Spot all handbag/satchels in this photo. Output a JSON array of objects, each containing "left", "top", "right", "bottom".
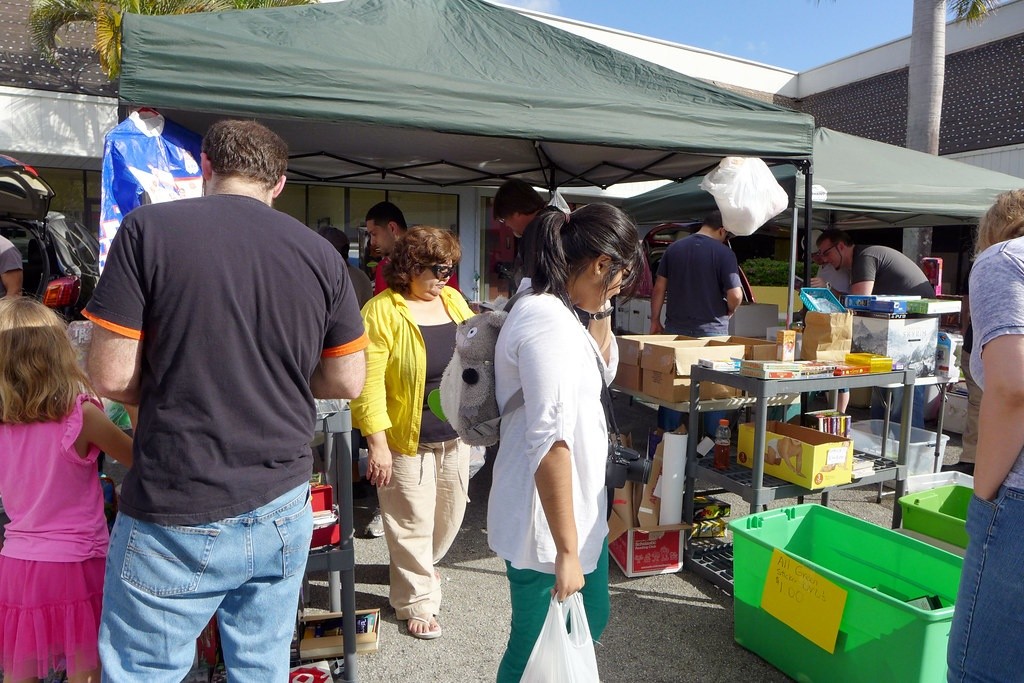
[{"left": 518, "top": 590, "right": 604, "bottom": 683}]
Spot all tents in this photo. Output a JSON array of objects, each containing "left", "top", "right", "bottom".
[
  {"left": 119, "top": 2, "right": 814, "bottom": 522},
  {"left": 618, "top": 124, "right": 1024, "bottom": 507}
]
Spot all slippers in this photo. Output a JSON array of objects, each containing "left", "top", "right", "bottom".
[{"left": 407, "top": 612, "right": 442, "bottom": 639}]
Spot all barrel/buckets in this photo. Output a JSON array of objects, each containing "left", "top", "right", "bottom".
[{"left": 924, "top": 383, "right": 943, "bottom": 421}]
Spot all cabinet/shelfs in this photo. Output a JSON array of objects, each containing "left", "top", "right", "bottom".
[
  {"left": 104, "top": 403, "right": 361, "bottom": 683},
  {"left": 684, "top": 364, "right": 916, "bottom": 597}
]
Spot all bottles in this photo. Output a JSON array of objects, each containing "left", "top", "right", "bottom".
[{"left": 713, "top": 419, "right": 731, "bottom": 469}]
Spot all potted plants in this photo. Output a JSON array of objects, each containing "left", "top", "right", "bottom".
[{"left": 741, "top": 259, "right": 819, "bottom": 314}]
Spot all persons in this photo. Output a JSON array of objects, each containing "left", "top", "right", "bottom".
[
  {"left": 946, "top": 189, "right": 1024, "bottom": 683},
  {"left": 809, "top": 228, "right": 941, "bottom": 430},
  {"left": 0, "top": 117, "right": 642, "bottom": 683},
  {"left": 650, "top": 214, "right": 745, "bottom": 443},
  {"left": 801, "top": 229, "right": 851, "bottom": 301}
]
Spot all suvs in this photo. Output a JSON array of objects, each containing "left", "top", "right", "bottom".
[{"left": 0, "top": 153, "right": 98, "bottom": 322}]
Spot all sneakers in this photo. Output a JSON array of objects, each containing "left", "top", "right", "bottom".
[{"left": 364, "top": 511, "right": 385, "bottom": 537}]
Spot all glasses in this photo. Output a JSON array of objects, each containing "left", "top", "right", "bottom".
[
  {"left": 819, "top": 241, "right": 838, "bottom": 257},
  {"left": 618, "top": 261, "right": 636, "bottom": 290},
  {"left": 498, "top": 214, "right": 509, "bottom": 223}
]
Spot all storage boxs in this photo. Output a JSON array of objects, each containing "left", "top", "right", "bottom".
[
  {"left": 310, "top": 485, "right": 341, "bottom": 548},
  {"left": 614, "top": 258, "right": 975, "bottom": 683}
]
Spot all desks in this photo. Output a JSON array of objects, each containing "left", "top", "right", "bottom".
[
  {"left": 877, "top": 375, "right": 965, "bottom": 504},
  {"left": 609, "top": 383, "right": 802, "bottom": 426}
]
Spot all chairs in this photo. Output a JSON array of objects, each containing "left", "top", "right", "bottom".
[
  {"left": 138, "top": 107, "right": 159, "bottom": 115},
  {"left": 28, "top": 239, "right": 43, "bottom": 286}
]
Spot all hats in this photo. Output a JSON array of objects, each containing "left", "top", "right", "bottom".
[{"left": 419, "top": 264, "right": 455, "bottom": 279}]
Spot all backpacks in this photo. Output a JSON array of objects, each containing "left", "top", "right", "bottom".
[{"left": 438, "top": 287, "right": 563, "bottom": 446}]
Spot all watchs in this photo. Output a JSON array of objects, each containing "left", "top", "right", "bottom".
[{"left": 588, "top": 306, "right": 613, "bottom": 320}]
